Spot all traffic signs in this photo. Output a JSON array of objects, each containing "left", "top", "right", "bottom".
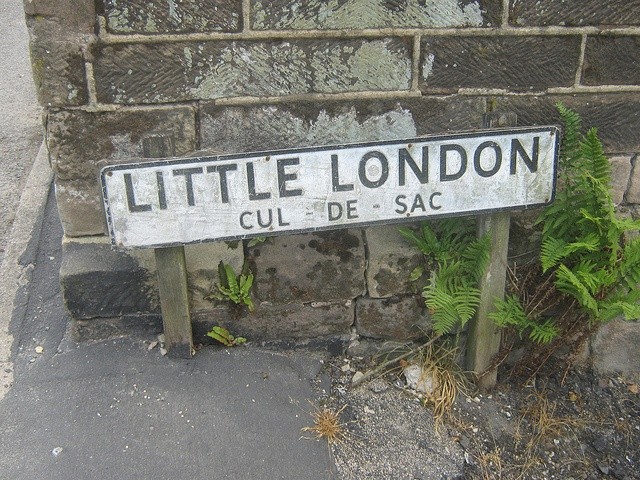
[{"left": 99, "top": 125, "right": 561, "bottom": 252}]
